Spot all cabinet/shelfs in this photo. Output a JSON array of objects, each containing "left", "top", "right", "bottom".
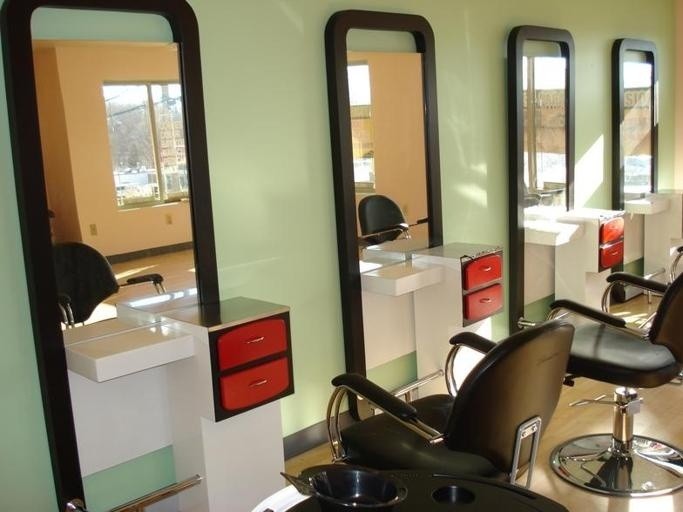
[
  {"left": 167, "top": 300, "right": 299, "bottom": 424},
  {"left": 564, "top": 210, "right": 627, "bottom": 276},
  {"left": 414, "top": 242, "right": 507, "bottom": 329}
]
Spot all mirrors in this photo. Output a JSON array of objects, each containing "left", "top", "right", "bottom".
[
  {"left": 507, "top": 22, "right": 577, "bottom": 229},
  {"left": 0, "top": 0, "right": 222, "bottom": 349},
  {"left": 610, "top": 37, "right": 661, "bottom": 209},
  {"left": 325, "top": 7, "right": 445, "bottom": 273}
]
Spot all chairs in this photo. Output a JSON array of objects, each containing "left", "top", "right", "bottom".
[
  {"left": 324, "top": 317, "right": 579, "bottom": 490},
  {"left": 520, "top": 268, "right": 683, "bottom": 499},
  {"left": 49, "top": 242, "right": 167, "bottom": 331},
  {"left": 358, "top": 195, "right": 430, "bottom": 247}
]
